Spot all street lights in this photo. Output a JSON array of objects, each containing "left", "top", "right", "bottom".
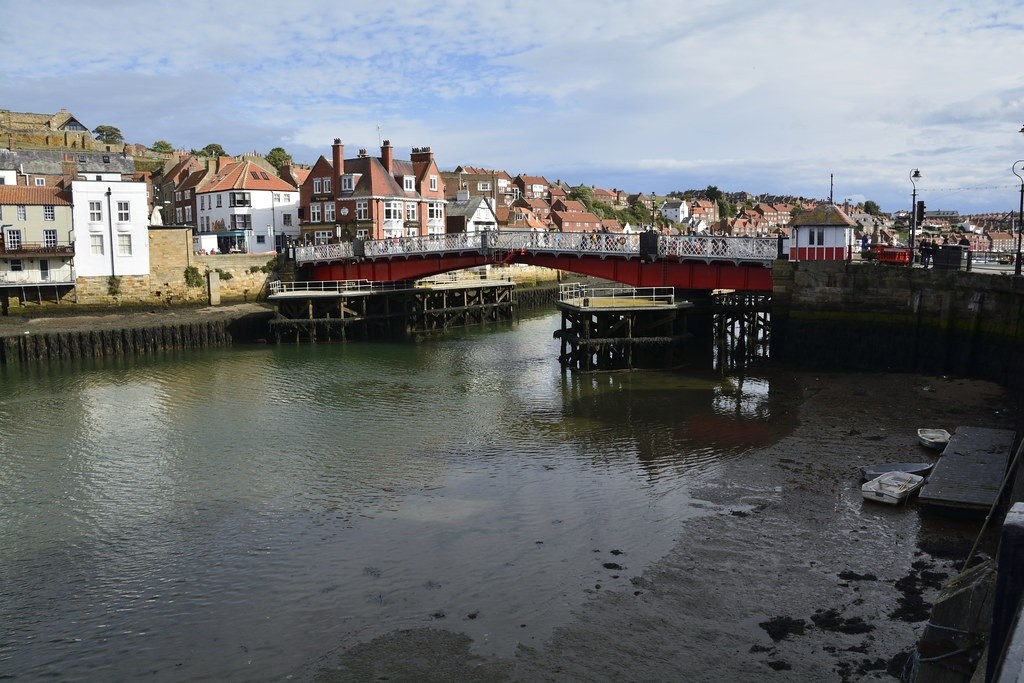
[
  {"left": 651, "top": 192, "right": 656, "bottom": 230},
  {"left": 910, "top": 168, "right": 922, "bottom": 262},
  {"left": 1012, "top": 160, "right": 1024, "bottom": 275}
]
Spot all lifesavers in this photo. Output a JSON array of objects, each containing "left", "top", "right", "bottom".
[
  {"left": 490, "top": 234, "right": 500, "bottom": 247},
  {"left": 618, "top": 237, "right": 626, "bottom": 246}
]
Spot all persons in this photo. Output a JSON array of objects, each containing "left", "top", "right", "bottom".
[
  {"left": 862, "top": 232, "right": 868, "bottom": 251},
  {"left": 530, "top": 227, "right": 621, "bottom": 251},
  {"left": 918, "top": 235, "right": 970, "bottom": 268},
  {"left": 300, "top": 234, "right": 398, "bottom": 259}
]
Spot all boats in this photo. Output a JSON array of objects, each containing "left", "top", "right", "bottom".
[
  {"left": 862, "top": 471, "right": 924, "bottom": 505},
  {"left": 918, "top": 428, "right": 951, "bottom": 448},
  {"left": 859, "top": 463, "right": 934, "bottom": 481}
]
